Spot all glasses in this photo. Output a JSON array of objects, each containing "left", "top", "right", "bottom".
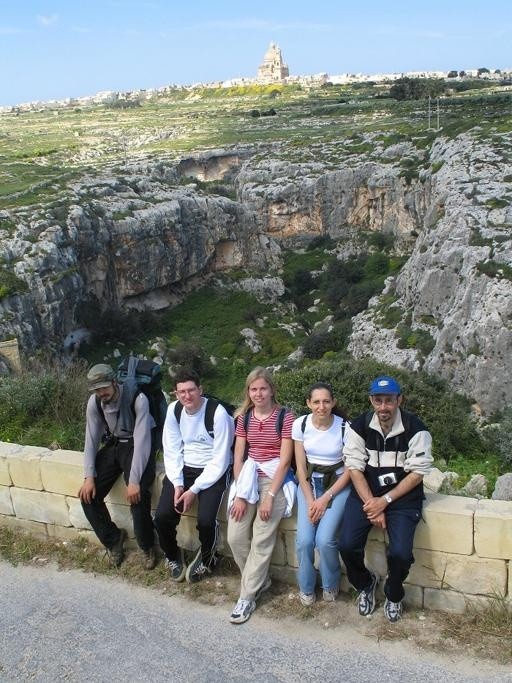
[{"left": 373, "top": 398, "right": 398, "bottom": 405}]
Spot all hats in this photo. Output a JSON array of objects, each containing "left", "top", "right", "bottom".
[
  {"left": 368, "top": 377, "right": 401, "bottom": 396},
  {"left": 87, "top": 363, "right": 117, "bottom": 392}
]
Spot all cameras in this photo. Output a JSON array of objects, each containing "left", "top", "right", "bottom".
[{"left": 378, "top": 472, "right": 397, "bottom": 488}]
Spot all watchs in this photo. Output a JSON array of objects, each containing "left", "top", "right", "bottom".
[
  {"left": 268, "top": 490, "right": 276, "bottom": 498},
  {"left": 384, "top": 494, "right": 393, "bottom": 503},
  {"left": 328, "top": 491, "right": 334, "bottom": 498}
]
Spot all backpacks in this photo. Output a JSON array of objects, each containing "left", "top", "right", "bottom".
[
  {"left": 174, "top": 394, "right": 237, "bottom": 438},
  {"left": 95, "top": 356, "right": 167, "bottom": 450}
]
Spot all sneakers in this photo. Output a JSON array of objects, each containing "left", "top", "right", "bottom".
[
  {"left": 229, "top": 599, "right": 257, "bottom": 624},
  {"left": 322, "top": 584, "right": 341, "bottom": 601},
  {"left": 108, "top": 527, "right": 128, "bottom": 567},
  {"left": 299, "top": 589, "right": 317, "bottom": 607},
  {"left": 185, "top": 546, "right": 218, "bottom": 584},
  {"left": 141, "top": 542, "right": 156, "bottom": 570},
  {"left": 382, "top": 574, "right": 403, "bottom": 623},
  {"left": 166, "top": 545, "right": 187, "bottom": 582},
  {"left": 356, "top": 570, "right": 381, "bottom": 616},
  {"left": 254, "top": 575, "right": 273, "bottom": 602}
]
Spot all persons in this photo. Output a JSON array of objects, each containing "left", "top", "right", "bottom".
[
  {"left": 155, "top": 370, "right": 235, "bottom": 584},
  {"left": 291, "top": 384, "right": 353, "bottom": 606},
  {"left": 342, "top": 377, "right": 434, "bottom": 622},
  {"left": 78, "top": 363, "right": 157, "bottom": 570},
  {"left": 226, "top": 366, "right": 296, "bottom": 624}
]
